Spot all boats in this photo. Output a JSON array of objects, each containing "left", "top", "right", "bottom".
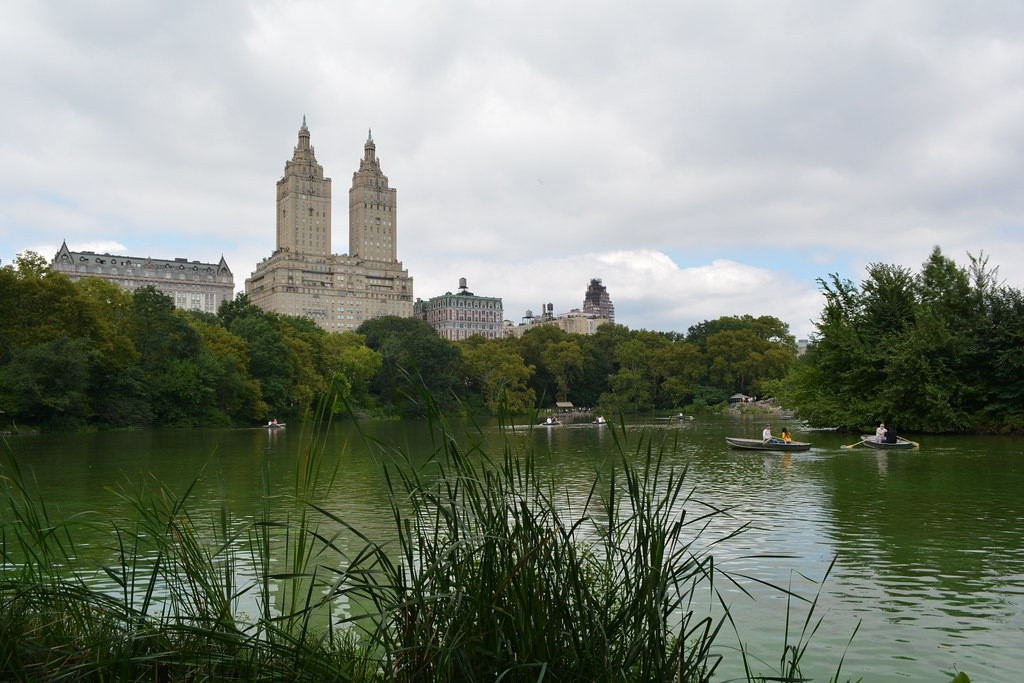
[
  {"left": 860, "top": 433, "right": 916, "bottom": 450},
  {"left": 543, "top": 420, "right": 563, "bottom": 426},
  {"left": 592, "top": 418, "right": 612, "bottom": 424},
  {"left": 262, "top": 423, "right": 288, "bottom": 427},
  {"left": 724, "top": 436, "right": 811, "bottom": 450}
]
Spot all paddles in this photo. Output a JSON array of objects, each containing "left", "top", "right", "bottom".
[
  {"left": 897, "top": 436, "right": 920, "bottom": 447},
  {"left": 761, "top": 437, "right": 772, "bottom": 446},
  {"left": 846, "top": 438, "right": 874, "bottom": 449}
]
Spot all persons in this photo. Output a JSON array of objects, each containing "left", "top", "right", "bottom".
[
  {"left": 781, "top": 428, "right": 791, "bottom": 441},
  {"left": 881, "top": 424, "right": 896, "bottom": 443},
  {"left": 547, "top": 416, "right": 557, "bottom": 424},
  {"left": 875, "top": 424, "right": 887, "bottom": 442},
  {"left": 763, "top": 424, "right": 777, "bottom": 443},
  {"left": 269, "top": 419, "right": 277, "bottom": 425}
]
